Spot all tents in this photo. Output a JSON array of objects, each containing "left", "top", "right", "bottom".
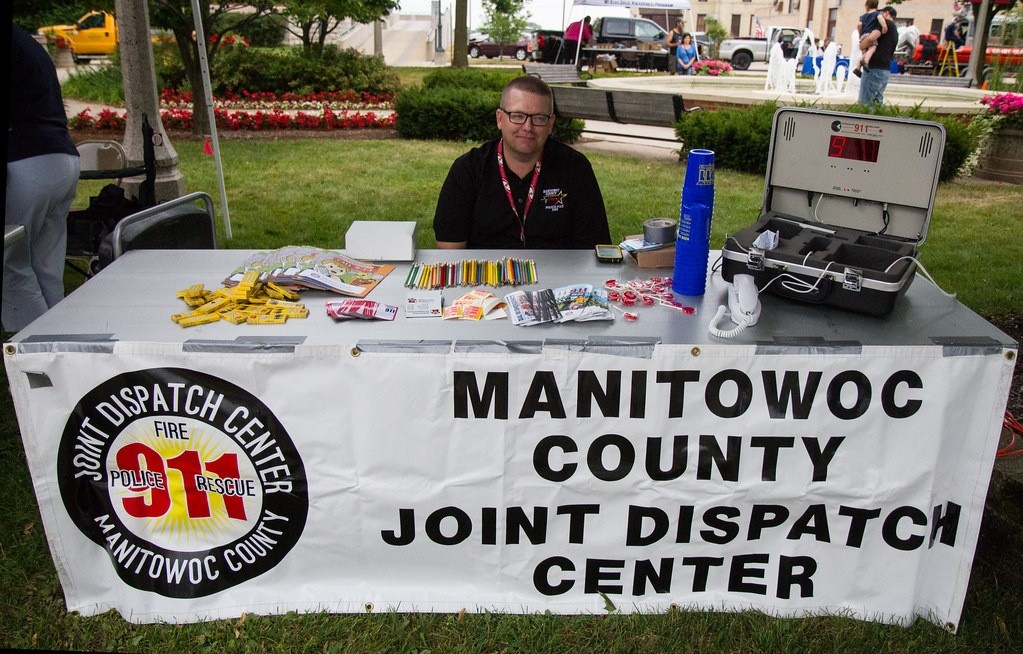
[{"left": 555, "top": 0, "right": 700, "bottom": 66}]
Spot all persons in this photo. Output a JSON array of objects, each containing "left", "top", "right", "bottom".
[
  {"left": 853, "top": 0, "right": 888, "bottom": 77},
  {"left": 561, "top": 295, "right": 586, "bottom": 310},
  {"left": 565, "top": 16, "right": 593, "bottom": 65},
  {"left": 432, "top": 77, "right": 611, "bottom": 250},
  {"left": 1, "top": 23, "right": 81, "bottom": 333},
  {"left": 807, "top": 38, "right": 830, "bottom": 55},
  {"left": 666, "top": 17, "right": 687, "bottom": 75},
  {"left": 944, "top": 22, "right": 963, "bottom": 55},
  {"left": 920, "top": 35, "right": 938, "bottom": 66},
  {"left": 676, "top": 32, "right": 697, "bottom": 76},
  {"left": 957, "top": 27, "right": 965, "bottom": 46},
  {"left": 897, "top": 59, "right": 905, "bottom": 74},
  {"left": 859, "top": 6, "right": 899, "bottom": 105},
  {"left": 790, "top": 34, "right": 801, "bottom": 58}
]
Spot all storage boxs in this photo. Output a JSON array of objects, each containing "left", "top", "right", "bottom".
[
  {"left": 627, "top": 235, "right": 679, "bottom": 269},
  {"left": 638, "top": 40, "right": 662, "bottom": 50},
  {"left": 594, "top": 59, "right": 618, "bottom": 74},
  {"left": 344, "top": 218, "right": 418, "bottom": 265}
]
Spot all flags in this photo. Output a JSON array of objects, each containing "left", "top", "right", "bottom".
[{"left": 755, "top": 18, "right": 762, "bottom": 37}]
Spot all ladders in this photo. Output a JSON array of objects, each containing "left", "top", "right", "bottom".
[{"left": 932, "top": 40, "right": 960, "bottom": 77}]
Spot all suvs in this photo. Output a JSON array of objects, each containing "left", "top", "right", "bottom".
[{"left": 912, "top": 12, "right": 1023, "bottom": 67}]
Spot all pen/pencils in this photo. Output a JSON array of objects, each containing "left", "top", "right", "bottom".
[{"left": 404, "top": 256, "right": 538, "bottom": 291}]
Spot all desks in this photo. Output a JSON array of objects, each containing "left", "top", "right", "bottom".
[
  {"left": 11, "top": 247, "right": 1018, "bottom": 636},
  {"left": 578, "top": 47, "right": 669, "bottom": 74}
]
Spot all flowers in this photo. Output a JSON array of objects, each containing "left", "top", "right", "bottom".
[{"left": 980, "top": 92, "right": 1023, "bottom": 128}]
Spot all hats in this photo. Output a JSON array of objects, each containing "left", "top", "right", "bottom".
[
  {"left": 676, "top": 17, "right": 687, "bottom": 24},
  {"left": 878, "top": 6, "right": 897, "bottom": 18}
]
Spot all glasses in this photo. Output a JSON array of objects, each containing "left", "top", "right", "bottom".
[{"left": 497, "top": 106, "right": 553, "bottom": 126}]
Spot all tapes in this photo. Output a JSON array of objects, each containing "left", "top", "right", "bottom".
[{"left": 643, "top": 218, "right": 677, "bottom": 243}]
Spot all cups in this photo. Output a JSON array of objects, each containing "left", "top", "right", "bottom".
[{"left": 672, "top": 149, "right": 713, "bottom": 296}]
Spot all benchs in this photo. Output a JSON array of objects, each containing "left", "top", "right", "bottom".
[{"left": 521, "top": 63, "right": 591, "bottom": 88}]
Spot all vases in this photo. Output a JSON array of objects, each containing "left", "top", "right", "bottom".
[{"left": 970, "top": 130, "right": 1023, "bottom": 183}]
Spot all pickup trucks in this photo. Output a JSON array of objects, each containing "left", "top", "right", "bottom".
[{"left": 718, "top": 26, "right": 821, "bottom": 71}]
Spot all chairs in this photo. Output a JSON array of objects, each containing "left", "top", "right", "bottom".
[
  {"left": 65, "top": 138, "right": 126, "bottom": 281},
  {"left": 65, "top": 111, "right": 157, "bottom": 279}
]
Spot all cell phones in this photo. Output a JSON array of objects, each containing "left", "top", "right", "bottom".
[{"left": 596, "top": 243, "right": 623, "bottom": 264}]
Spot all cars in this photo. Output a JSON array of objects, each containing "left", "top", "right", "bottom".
[
  {"left": 466, "top": 14, "right": 717, "bottom": 72},
  {"left": 35, "top": 8, "right": 164, "bottom": 66}
]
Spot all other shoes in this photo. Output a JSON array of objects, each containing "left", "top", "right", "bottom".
[
  {"left": 860, "top": 59, "right": 869, "bottom": 73},
  {"left": 853, "top": 69, "right": 862, "bottom": 78}
]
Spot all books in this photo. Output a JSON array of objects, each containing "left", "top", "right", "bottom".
[{"left": 219, "top": 245, "right": 396, "bottom": 298}]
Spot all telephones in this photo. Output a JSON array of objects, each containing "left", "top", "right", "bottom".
[{"left": 727, "top": 274, "right": 762, "bottom": 326}]
